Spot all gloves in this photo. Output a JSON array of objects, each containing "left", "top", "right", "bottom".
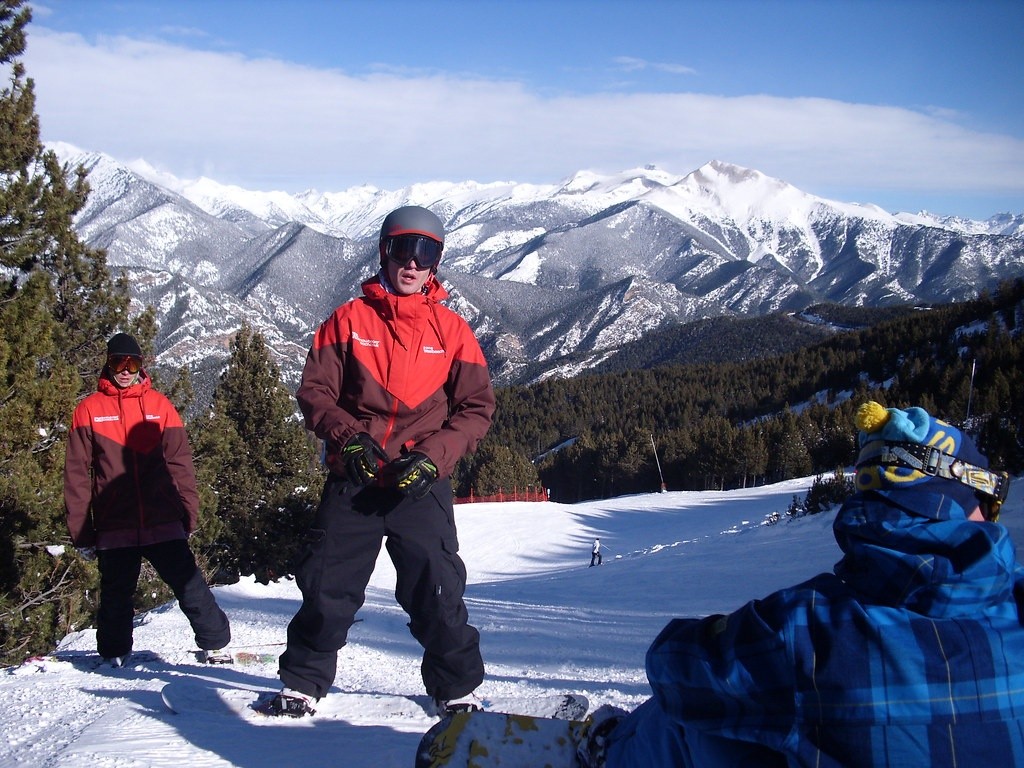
[
  {"left": 76, "top": 544, "right": 96, "bottom": 561},
  {"left": 392, "top": 451, "right": 439, "bottom": 500},
  {"left": 342, "top": 431, "right": 386, "bottom": 485}
]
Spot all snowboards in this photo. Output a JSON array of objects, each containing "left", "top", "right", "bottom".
[
  {"left": 415, "top": 712, "right": 591, "bottom": 768},
  {"left": 25, "top": 653, "right": 279, "bottom": 672},
  {"left": 161, "top": 683, "right": 588, "bottom": 726}
]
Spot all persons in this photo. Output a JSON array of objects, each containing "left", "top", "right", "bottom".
[
  {"left": 573, "top": 402, "right": 1023, "bottom": 768},
  {"left": 268, "top": 205, "right": 497, "bottom": 718},
  {"left": 589, "top": 538, "right": 603, "bottom": 567},
  {"left": 64, "top": 333, "right": 233, "bottom": 674}
]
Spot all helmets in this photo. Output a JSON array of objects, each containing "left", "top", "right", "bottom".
[{"left": 377, "top": 206, "right": 445, "bottom": 274}]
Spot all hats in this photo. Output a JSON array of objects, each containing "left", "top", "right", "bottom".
[
  {"left": 855, "top": 406, "right": 988, "bottom": 518},
  {"left": 106, "top": 333, "right": 141, "bottom": 355}
]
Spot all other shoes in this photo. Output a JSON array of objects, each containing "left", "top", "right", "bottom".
[
  {"left": 576, "top": 701, "right": 629, "bottom": 768},
  {"left": 201, "top": 647, "right": 233, "bottom": 664},
  {"left": 267, "top": 686, "right": 315, "bottom": 716},
  {"left": 433, "top": 692, "right": 486, "bottom": 720}
]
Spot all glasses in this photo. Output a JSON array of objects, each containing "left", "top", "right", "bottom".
[
  {"left": 382, "top": 234, "right": 440, "bottom": 269},
  {"left": 984, "top": 468, "right": 1010, "bottom": 515},
  {"left": 106, "top": 355, "right": 142, "bottom": 373}
]
